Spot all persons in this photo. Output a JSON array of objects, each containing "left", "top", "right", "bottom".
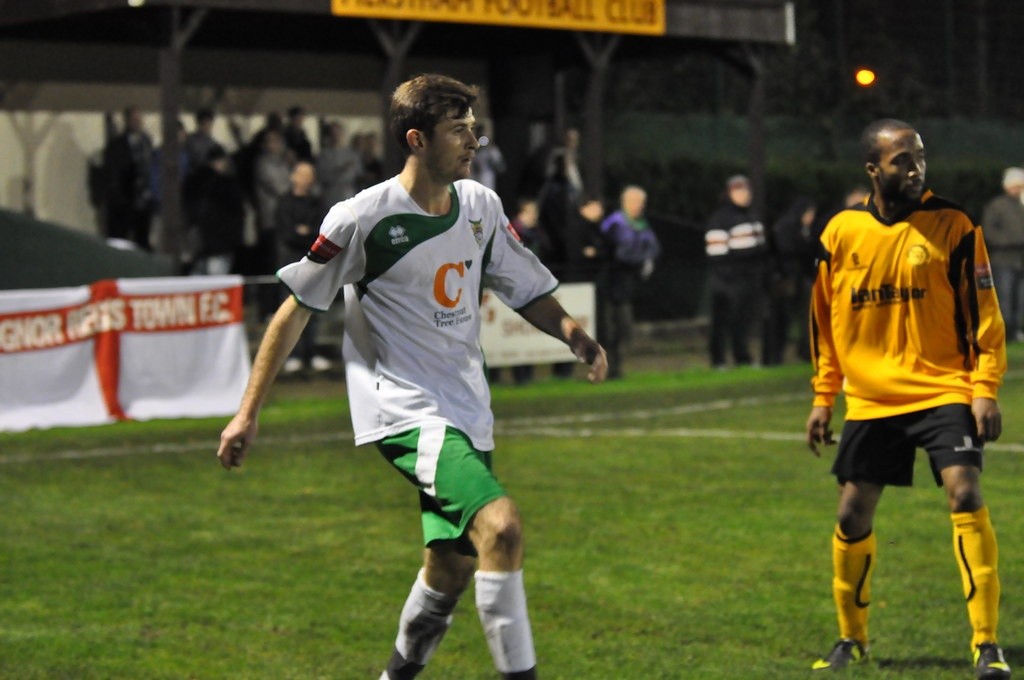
[
  {"left": 508, "top": 196, "right": 551, "bottom": 385},
  {"left": 980, "top": 167, "right": 1024, "bottom": 341},
  {"left": 804, "top": 116, "right": 1010, "bottom": 680},
  {"left": 762, "top": 197, "right": 816, "bottom": 366},
  {"left": 543, "top": 122, "right": 595, "bottom": 203},
  {"left": 101, "top": 105, "right": 386, "bottom": 372},
  {"left": 705, "top": 174, "right": 765, "bottom": 369},
  {"left": 215, "top": 72, "right": 610, "bottom": 680},
  {"left": 552, "top": 194, "right": 615, "bottom": 381},
  {"left": 599, "top": 183, "right": 660, "bottom": 378},
  {"left": 467, "top": 117, "right": 507, "bottom": 191},
  {"left": 843, "top": 182, "right": 870, "bottom": 207}
]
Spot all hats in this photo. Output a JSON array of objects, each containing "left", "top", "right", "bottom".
[{"left": 727, "top": 175, "right": 751, "bottom": 191}]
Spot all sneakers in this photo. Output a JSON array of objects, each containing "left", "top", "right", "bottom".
[
  {"left": 811, "top": 637, "right": 870, "bottom": 670},
  {"left": 971, "top": 641, "right": 1012, "bottom": 680}
]
